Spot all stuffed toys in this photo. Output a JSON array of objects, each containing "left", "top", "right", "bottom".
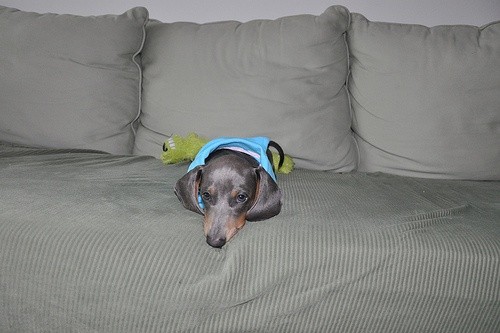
[{"left": 160, "top": 131, "right": 295, "bottom": 174}]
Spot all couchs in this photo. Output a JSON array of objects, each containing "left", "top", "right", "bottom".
[{"left": 0, "top": 5, "right": 500, "bottom": 333}]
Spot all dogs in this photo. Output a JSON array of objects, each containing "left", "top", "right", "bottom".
[{"left": 173, "top": 136, "right": 285, "bottom": 248}]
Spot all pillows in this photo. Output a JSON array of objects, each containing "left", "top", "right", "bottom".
[
  {"left": 0, "top": 6, "right": 150, "bottom": 157},
  {"left": 132, "top": 5, "right": 359, "bottom": 174},
  {"left": 346, "top": 12, "right": 500, "bottom": 181}
]
